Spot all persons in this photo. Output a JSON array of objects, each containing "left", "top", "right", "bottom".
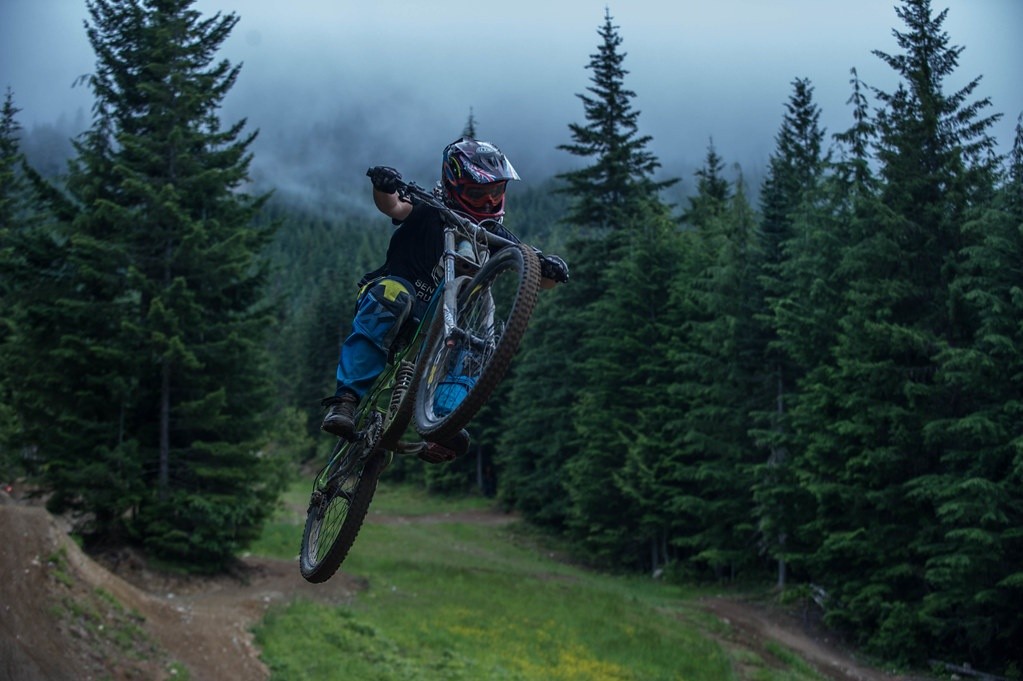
[{"left": 321, "top": 138, "right": 569, "bottom": 464}]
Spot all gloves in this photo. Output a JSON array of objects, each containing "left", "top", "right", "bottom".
[
  {"left": 540, "top": 255, "right": 570, "bottom": 284},
  {"left": 370, "top": 165, "right": 402, "bottom": 194}
]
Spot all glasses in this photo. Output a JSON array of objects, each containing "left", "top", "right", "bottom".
[{"left": 457, "top": 180, "right": 506, "bottom": 208}]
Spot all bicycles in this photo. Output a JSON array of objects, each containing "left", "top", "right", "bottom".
[{"left": 295, "top": 166, "right": 542, "bottom": 582}]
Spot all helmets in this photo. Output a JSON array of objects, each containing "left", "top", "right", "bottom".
[{"left": 441, "top": 138, "right": 521, "bottom": 223}]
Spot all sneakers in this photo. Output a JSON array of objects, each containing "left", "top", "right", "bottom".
[
  {"left": 320, "top": 385, "right": 360, "bottom": 437},
  {"left": 416, "top": 428, "right": 470, "bottom": 464}
]
